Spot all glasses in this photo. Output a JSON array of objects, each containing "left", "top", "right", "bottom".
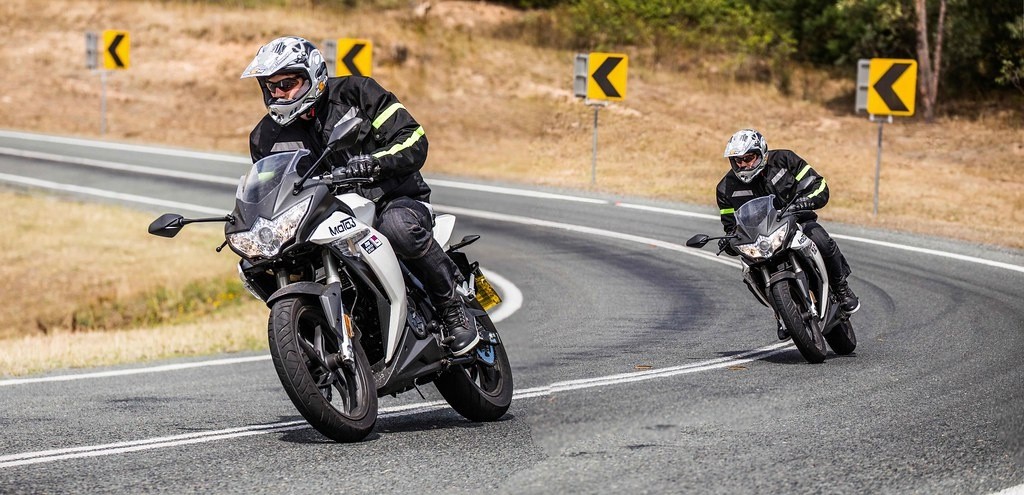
[
  {"left": 265, "top": 74, "right": 301, "bottom": 93},
  {"left": 735, "top": 153, "right": 756, "bottom": 164}
]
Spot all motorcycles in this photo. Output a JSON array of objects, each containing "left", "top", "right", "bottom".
[
  {"left": 148, "top": 116, "right": 513, "bottom": 443},
  {"left": 686, "top": 176, "right": 857, "bottom": 363}
]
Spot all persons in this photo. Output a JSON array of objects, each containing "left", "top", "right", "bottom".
[
  {"left": 249, "top": 36, "right": 480, "bottom": 357},
  {"left": 716, "top": 128, "right": 861, "bottom": 343}
]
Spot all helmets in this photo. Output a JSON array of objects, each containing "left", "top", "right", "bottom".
[
  {"left": 724, "top": 129, "right": 769, "bottom": 183},
  {"left": 240, "top": 35, "right": 328, "bottom": 127}
]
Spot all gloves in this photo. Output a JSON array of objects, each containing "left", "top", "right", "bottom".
[
  {"left": 346, "top": 154, "right": 374, "bottom": 178},
  {"left": 718, "top": 236, "right": 731, "bottom": 252},
  {"left": 796, "top": 197, "right": 810, "bottom": 209}
]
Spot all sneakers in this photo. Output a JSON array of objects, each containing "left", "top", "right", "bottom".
[
  {"left": 832, "top": 280, "right": 860, "bottom": 315},
  {"left": 440, "top": 303, "right": 480, "bottom": 356},
  {"left": 777, "top": 321, "right": 792, "bottom": 342}
]
